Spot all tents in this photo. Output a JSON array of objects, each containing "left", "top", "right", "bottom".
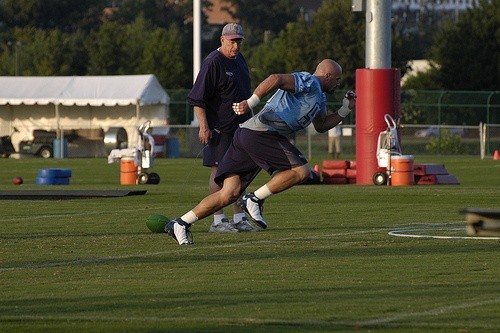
[{"left": 0, "top": 74, "right": 171, "bottom": 157}]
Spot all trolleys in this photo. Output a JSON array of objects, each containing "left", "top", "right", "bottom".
[
  {"left": 371, "top": 114, "right": 404, "bottom": 186},
  {"left": 134, "top": 119, "right": 161, "bottom": 185}
]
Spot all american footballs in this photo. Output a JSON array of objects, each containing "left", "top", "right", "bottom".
[{"left": 13, "top": 177, "right": 23, "bottom": 184}]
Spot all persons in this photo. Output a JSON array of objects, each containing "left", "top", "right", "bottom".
[
  {"left": 186, "top": 23, "right": 253, "bottom": 233},
  {"left": 165, "top": 59, "right": 357, "bottom": 245},
  {"left": 328, "top": 121, "right": 342, "bottom": 159}
]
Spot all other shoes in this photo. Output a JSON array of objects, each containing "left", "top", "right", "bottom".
[
  {"left": 230, "top": 217, "right": 262, "bottom": 231},
  {"left": 209, "top": 218, "right": 239, "bottom": 232}
]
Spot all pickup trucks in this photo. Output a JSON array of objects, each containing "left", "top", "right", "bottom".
[{"left": 20, "top": 133, "right": 102, "bottom": 158}]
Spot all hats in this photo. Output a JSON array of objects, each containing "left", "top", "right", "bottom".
[{"left": 221, "top": 23, "right": 246, "bottom": 40}]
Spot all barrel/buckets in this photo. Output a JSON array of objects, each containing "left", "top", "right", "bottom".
[
  {"left": 390, "top": 155, "right": 416, "bottom": 185},
  {"left": 119, "top": 157, "right": 138, "bottom": 185},
  {"left": 53, "top": 139, "right": 67, "bottom": 159},
  {"left": 166, "top": 138, "right": 180, "bottom": 159}
]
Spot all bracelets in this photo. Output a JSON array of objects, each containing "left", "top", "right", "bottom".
[
  {"left": 247, "top": 94, "right": 261, "bottom": 109},
  {"left": 338, "top": 106, "right": 351, "bottom": 118}
]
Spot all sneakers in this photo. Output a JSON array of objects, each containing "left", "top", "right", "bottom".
[
  {"left": 237, "top": 191, "right": 267, "bottom": 229},
  {"left": 164, "top": 217, "right": 195, "bottom": 246}
]
